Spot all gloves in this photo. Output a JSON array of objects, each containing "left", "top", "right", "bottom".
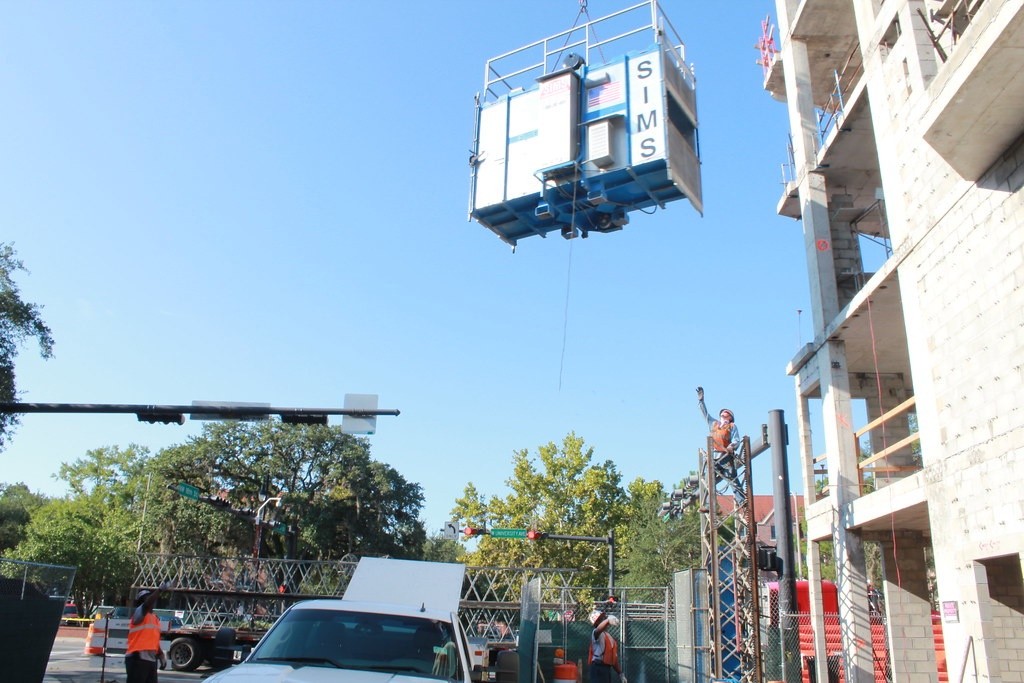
[
  {"left": 727, "top": 443, "right": 734, "bottom": 454},
  {"left": 619, "top": 673, "right": 627, "bottom": 683},
  {"left": 608, "top": 614, "right": 620, "bottom": 628},
  {"left": 159, "top": 656, "right": 167, "bottom": 670},
  {"left": 696, "top": 387, "right": 704, "bottom": 401}
]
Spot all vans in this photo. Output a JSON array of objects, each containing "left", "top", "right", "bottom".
[{"left": 60, "top": 604, "right": 80, "bottom": 625}]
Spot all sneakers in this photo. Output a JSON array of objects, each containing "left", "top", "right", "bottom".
[
  {"left": 697, "top": 506, "right": 722, "bottom": 515},
  {"left": 744, "top": 510, "right": 753, "bottom": 524}
]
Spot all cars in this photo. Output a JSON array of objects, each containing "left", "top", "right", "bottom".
[{"left": 202, "top": 557, "right": 476, "bottom": 683}]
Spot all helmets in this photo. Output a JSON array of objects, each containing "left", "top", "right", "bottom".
[
  {"left": 719, "top": 409, "right": 734, "bottom": 423},
  {"left": 589, "top": 610, "right": 606, "bottom": 627},
  {"left": 136, "top": 590, "right": 151, "bottom": 602}
]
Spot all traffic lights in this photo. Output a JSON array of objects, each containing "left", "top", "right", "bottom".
[
  {"left": 526, "top": 531, "right": 541, "bottom": 540},
  {"left": 758, "top": 545, "right": 778, "bottom": 573},
  {"left": 464, "top": 526, "right": 474, "bottom": 536}
]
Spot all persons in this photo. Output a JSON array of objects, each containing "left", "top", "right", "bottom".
[
  {"left": 696, "top": 386, "right": 750, "bottom": 524},
  {"left": 587, "top": 609, "right": 629, "bottom": 683},
  {"left": 124, "top": 582, "right": 167, "bottom": 682}
]
